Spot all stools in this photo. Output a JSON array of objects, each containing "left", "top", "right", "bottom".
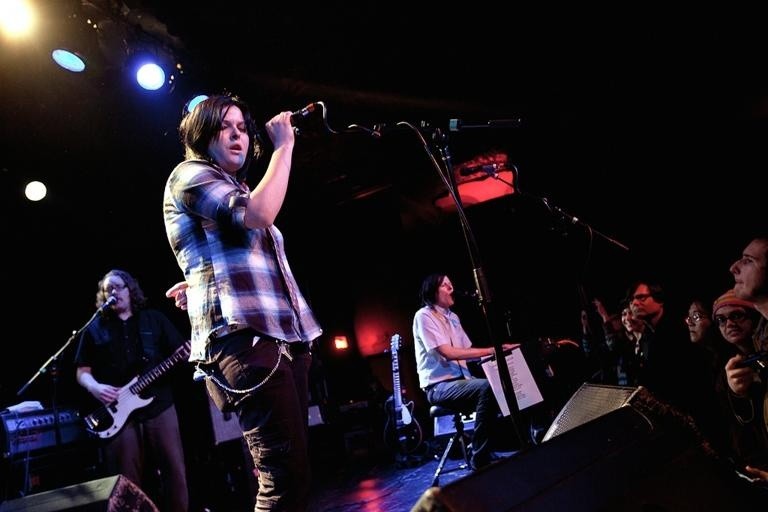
[{"left": 428, "top": 405, "right": 473, "bottom": 487}]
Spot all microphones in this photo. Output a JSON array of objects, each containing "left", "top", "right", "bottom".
[
  {"left": 254, "top": 97, "right": 318, "bottom": 134},
  {"left": 95, "top": 294, "right": 120, "bottom": 316},
  {"left": 460, "top": 160, "right": 506, "bottom": 177}
]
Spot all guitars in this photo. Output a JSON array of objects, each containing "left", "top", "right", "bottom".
[
  {"left": 381, "top": 333, "right": 423, "bottom": 455},
  {"left": 74, "top": 336, "right": 192, "bottom": 440}
]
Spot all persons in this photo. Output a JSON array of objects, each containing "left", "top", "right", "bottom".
[
  {"left": 576, "top": 296, "right": 631, "bottom": 387},
  {"left": 74, "top": 268, "right": 191, "bottom": 512},
  {"left": 161, "top": 89, "right": 322, "bottom": 512},
  {"left": 410, "top": 273, "right": 511, "bottom": 473},
  {"left": 622, "top": 278, "right": 685, "bottom": 399},
  {"left": 725, "top": 231, "right": 767, "bottom": 511},
  {"left": 709, "top": 288, "right": 759, "bottom": 352},
  {"left": 682, "top": 289, "right": 712, "bottom": 348},
  {"left": 615, "top": 297, "right": 647, "bottom": 389}
]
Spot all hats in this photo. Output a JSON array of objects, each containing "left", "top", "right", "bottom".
[{"left": 711, "top": 288, "right": 754, "bottom": 320}]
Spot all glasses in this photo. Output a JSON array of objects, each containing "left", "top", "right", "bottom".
[
  {"left": 628, "top": 293, "right": 654, "bottom": 303},
  {"left": 685, "top": 313, "right": 707, "bottom": 324},
  {"left": 716, "top": 312, "right": 755, "bottom": 324},
  {"left": 103, "top": 284, "right": 129, "bottom": 294}
]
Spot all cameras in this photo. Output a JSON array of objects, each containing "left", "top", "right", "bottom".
[{"left": 735, "top": 354, "right": 762, "bottom": 369}]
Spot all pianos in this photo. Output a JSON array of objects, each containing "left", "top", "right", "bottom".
[{"left": 0, "top": 404, "right": 78, "bottom": 459}]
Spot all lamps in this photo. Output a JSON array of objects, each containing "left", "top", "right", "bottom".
[{"left": 47, "top": 29, "right": 103, "bottom": 75}]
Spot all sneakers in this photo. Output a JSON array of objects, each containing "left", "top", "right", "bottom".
[{"left": 469, "top": 440, "right": 506, "bottom": 471}]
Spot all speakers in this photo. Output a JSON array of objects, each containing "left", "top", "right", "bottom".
[{"left": 540, "top": 381, "right": 663, "bottom": 461}]
